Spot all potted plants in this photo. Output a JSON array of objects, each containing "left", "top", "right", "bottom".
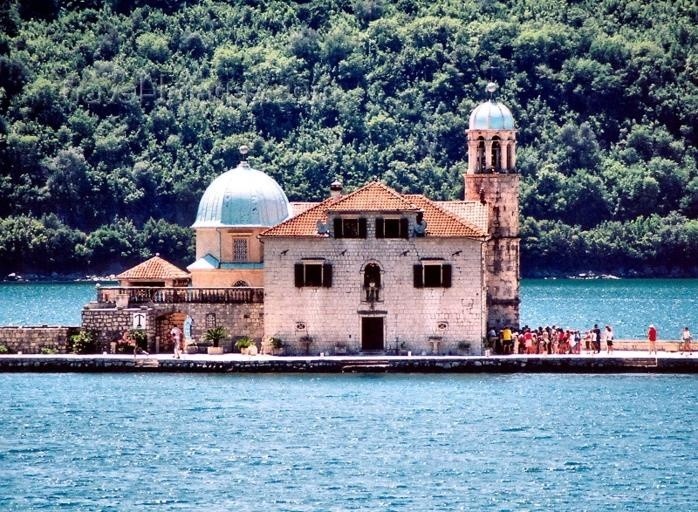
[
  {"left": 481, "top": 337, "right": 491, "bottom": 356},
  {"left": 401, "top": 341, "right": 408, "bottom": 356},
  {"left": 334, "top": 341, "right": 346, "bottom": 354},
  {"left": 457, "top": 340, "right": 471, "bottom": 355},
  {"left": 187, "top": 326, "right": 286, "bottom": 355}
]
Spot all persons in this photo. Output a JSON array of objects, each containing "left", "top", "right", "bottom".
[
  {"left": 170, "top": 324, "right": 183, "bottom": 359},
  {"left": 487, "top": 324, "right": 614, "bottom": 354},
  {"left": 411, "top": 213, "right": 428, "bottom": 237},
  {"left": 647, "top": 325, "right": 657, "bottom": 355},
  {"left": 680, "top": 327, "right": 693, "bottom": 355},
  {"left": 131, "top": 279, "right": 192, "bottom": 302},
  {"left": 171, "top": 333, "right": 176, "bottom": 345},
  {"left": 314, "top": 215, "right": 329, "bottom": 234}
]
